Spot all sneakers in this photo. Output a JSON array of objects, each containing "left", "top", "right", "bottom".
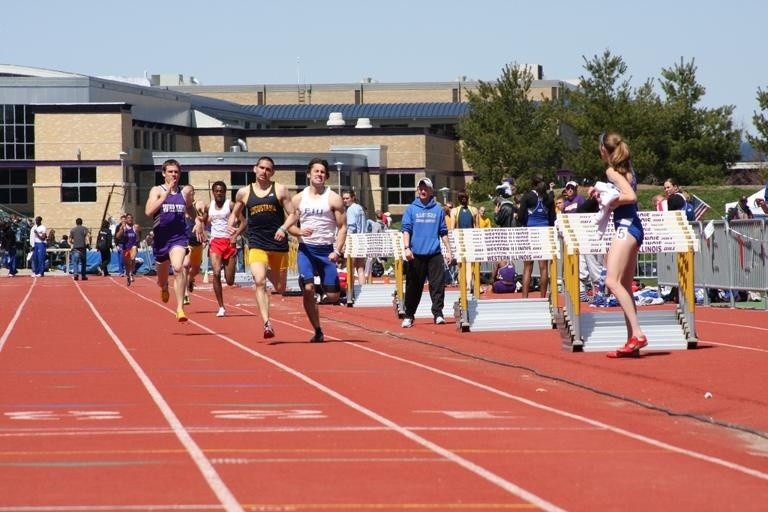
[
  {"left": 309, "top": 332, "right": 323, "bottom": 343},
  {"left": 400, "top": 317, "right": 414, "bottom": 328},
  {"left": 188, "top": 279, "right": 196, "bottom": 293},
  {"left": 183, "top": 296, "right": 190, "bottom": 304},
  {"left": 216, "top": 306, "right": 227, "bottom": 317},
  {"left": 263, "top": 320, "right": 275, "bottom": 339},
  {"left": 434, "top": 314, "right": 446, "bottom": 325},
  {"left": 7, "top": 265, "right": 135, "bottom": 289},
  {"left": 616, "top": 335, "right": 649, "bottom": 354},
  {"left": 176, "top": 309, "right": 188, "bottom": 322},
  {"left": 606, "top": 349, "right": 640, "bottom": 359},
  {"left": 161, "top": 284, "right": 169, "bottom": 303}
]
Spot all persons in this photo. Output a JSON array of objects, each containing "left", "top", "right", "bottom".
[
  {"left": 286, "top": 158, "right": 348, "bottom": 343},
  {"left": 203, "top": 181, "right": 247, "bottom": 316},
  {"left": 227, "top": 157, "right": 300, "bottom": 338},
  {"left": 401, "top": 177, "right": 452, "bottom": 328},
  {"left": 182, "top": 184, "right": 205, "bottom": 305},
  {"left": 342, "top": 173, "right": 768, "bottom": 303},
  {"left": 587, "top": 130, "right": 648, "bottom": 356},
  {"left": 145, "top": 159, "right": 207, "bottom": 321},
  {"left": 0, "top": 213, "right": 155, "bottom": 285}
]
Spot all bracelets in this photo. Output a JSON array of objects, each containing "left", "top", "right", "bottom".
[
  {"left": 277, "top": 227, "right": 286, "bottom": 233},
  {"left": 334, "top": 250, "right": 341, "bottom": 256}
]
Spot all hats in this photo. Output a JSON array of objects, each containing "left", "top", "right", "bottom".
[
  {"left": 565, "top": 180, "right": 579, "bottom": 188},
  {"left": 416, "top": 176, "right": 434, "bottom": 190},
  {"left": 740, "top": 195, "right": 748, "bottom": 202}
]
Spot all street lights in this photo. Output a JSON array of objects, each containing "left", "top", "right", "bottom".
[
  {"left": 439, "top": 186, "right": 450, "bottom": 208},
  {"left": 334, "top": 160, "right": 344, "bottom": 198}
]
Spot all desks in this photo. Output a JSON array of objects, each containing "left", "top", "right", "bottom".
[{"left": 43, "top": 246, "right": 72, "bottom": 275}]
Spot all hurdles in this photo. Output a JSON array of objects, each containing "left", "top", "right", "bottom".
[
  {"left": 346, "top": 225, "right": 560, "bottom": 332},
  {"left": 554, "top": 210, "right": 699, "bottom": 353}
]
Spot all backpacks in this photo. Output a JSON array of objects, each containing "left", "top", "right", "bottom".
[{"left": 674, "top": 193, "right": 696, "bottom": 222}]
